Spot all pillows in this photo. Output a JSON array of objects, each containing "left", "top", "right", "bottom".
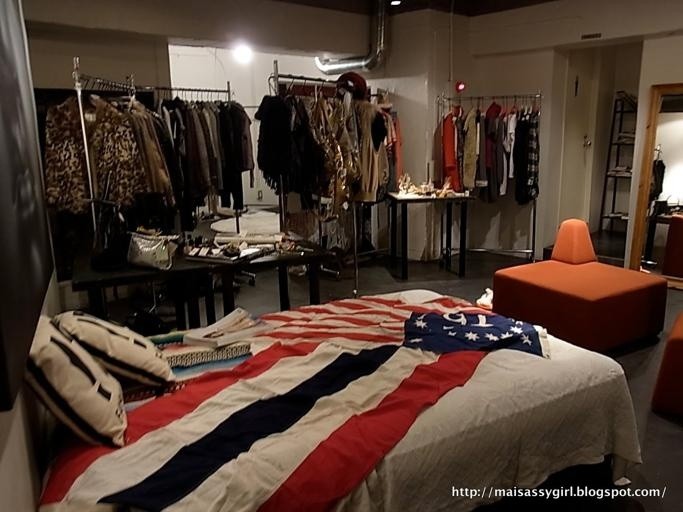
[
  {"left": 48, "top": 309, "right": 177, "bottom": 391},
  {"left": 22, "top": 315, "right": 129, "bottom": 451}
]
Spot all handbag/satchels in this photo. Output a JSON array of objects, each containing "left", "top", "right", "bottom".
[{"left": 127, "top": 232, "right": 179, "bottom": 271}]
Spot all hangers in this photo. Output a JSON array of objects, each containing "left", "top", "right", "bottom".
[
  {"left": 71, "top": 69, "right": 243, "bottom": 115},
  {"left": 267, "top": 73, "right": 385, "bottom": 106},
  {"left": 454, "top": 93, "right": 540, "bottom": 120}
]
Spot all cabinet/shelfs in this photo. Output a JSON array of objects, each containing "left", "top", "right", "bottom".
[{"left": 596, "top": 97, "right": 636, "bottom": 236}]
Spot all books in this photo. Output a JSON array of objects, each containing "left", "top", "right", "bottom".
[{"left": 181, "top": 307, "right": 273, "bottom": 348}]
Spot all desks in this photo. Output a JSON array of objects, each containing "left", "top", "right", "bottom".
[
  {"left": 387, "top": 191, "right": 477, "bottom": 280},
  {"left": 70, "top": 231, "right": 326, "bottom": 326}
]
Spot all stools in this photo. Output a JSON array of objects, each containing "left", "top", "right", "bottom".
[{"left": 648, "top": 312, "right": 682, "bottom": 422}]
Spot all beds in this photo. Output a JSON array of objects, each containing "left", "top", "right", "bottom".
[{"left": 38, "top": 286, "right": 645, "bottom": 511}]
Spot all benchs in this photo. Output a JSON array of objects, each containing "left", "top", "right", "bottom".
[{"left": 492, "top": 219, "right": 668, "bottom": 352}]
